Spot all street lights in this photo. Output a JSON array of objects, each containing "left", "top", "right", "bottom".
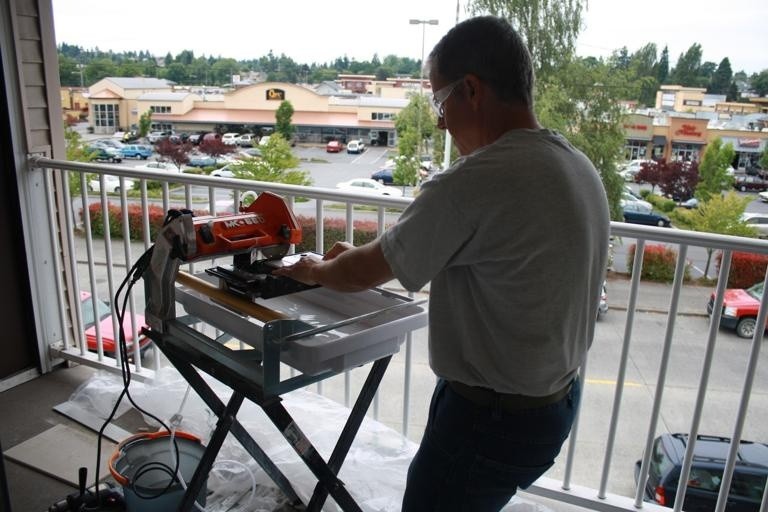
[{"left": 408, "top": 19, "right": 440, "bottom": 188}]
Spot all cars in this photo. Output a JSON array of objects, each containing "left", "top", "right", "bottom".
[
  {"left": 616, "top": 159, "right": 659, "bottom": 184},
  {"left": 683, "top": 197, "right": 698, "bottom": 208},
  {"left": 87, "top": 131, "right": 270, "bottom": 191},
  {"left": 726, "top": 165, "right": 768, "bottom": 237},
  {"left": 79, "top": 292, "right": 152, "bottom": 362},
  {"left": 336, "top": 154, "right": 433, "bottom": 198},
  {"left": 707, "top": 280, "right": 768, "bottom": 339},
  {"left": 618, "top": 192, "right": 670, "bottom": 228},
  {"left": 326, "top": 139, "right": 365, "bottom": 152},
  {"left": 635, "top": 432, "right": 768, "bottom": 512}
]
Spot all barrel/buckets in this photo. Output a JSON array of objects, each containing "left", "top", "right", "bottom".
[{"left": 109, "top": 430, "right": 211, "bottom": 512}]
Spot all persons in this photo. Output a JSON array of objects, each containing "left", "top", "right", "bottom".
[{"left": 272, "top": 15, "right": 610, "bottom": 512}]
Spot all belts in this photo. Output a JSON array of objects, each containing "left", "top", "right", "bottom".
[{"left": 449, "top": 383, "right": 572, "bottom": 411}]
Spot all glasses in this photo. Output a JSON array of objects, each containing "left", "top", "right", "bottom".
[{"left": 429, "top": 79, "right": 467, "bottom": 118}]
